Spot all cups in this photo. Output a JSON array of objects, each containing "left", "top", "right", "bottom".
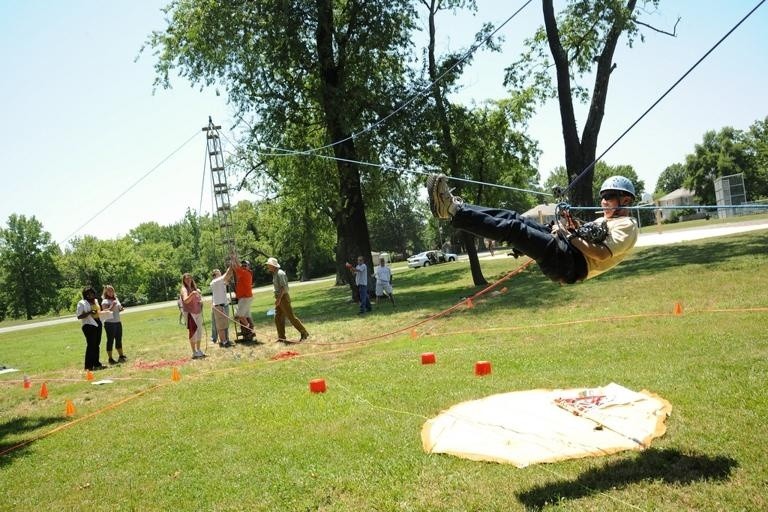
[
  {"left": 242, "top": 264, "right": 247, "bottom": 267},
  {"left": 598, "top": 193, "right": 624, "bottom": 202}
]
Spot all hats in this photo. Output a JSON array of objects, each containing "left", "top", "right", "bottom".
[{"left": 407, "top": 250, "right": 458, "bottom": 269}]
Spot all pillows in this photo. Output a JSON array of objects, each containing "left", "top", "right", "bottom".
[{"left": 599, "top": 174, "right": 636, "bottom": 202}]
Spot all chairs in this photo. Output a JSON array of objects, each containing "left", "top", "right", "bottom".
[
  {"left": 299, "top": 332, "right": 309, "bottom": 342},
  {"left": 108, "top": 358, "right": 118, "bottom": 364},
  {"left": 84, "top": 365, "right": 108, "bottom": 371},
  {"left": 426, "top": 171, "right": 455, "bottom": 219},
  {"left": 118, "top": 355, "right": 127, "bottom": 363},
  {"left": 276, "top": 337, "right": 286, "bottom": 342},
  {"left": 219, "top": 340, "right": 235, "bottom": 347},
  {"left": 191, "top": 350, "right": 207, "bottom": 359}
]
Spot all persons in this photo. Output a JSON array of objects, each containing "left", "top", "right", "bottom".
[
  {"left": 101, "top": 284, "right": 128, "bottom": 364},
  {"left": 427, "top": 173, "right": 639, "bottom": 284},
  {"left": 369, "top": 257, "right": 399, "bottom": 309},
  {"left": 76, "top": 287, "right": 108, "bottom": 371},
  {"left": 210, "top": 296, "right": 233, "bottom": 344},
  {"left": 430, "top": 254, "right": 438, "bottom": 264},
  {"left": 230, "top": 250, "right": 257, "bottom": 342},
  {"left": 210, "top": 263, "right": 234, "bottom": 347},
  {"left": 344, "top": 254, "right": 373, "bottom": 316},
  {"left": 180, "top": 272, "right": 206, "bottom": 358},
  {"left": 264, "top": 257, "right": 310, "bottom": 344}
]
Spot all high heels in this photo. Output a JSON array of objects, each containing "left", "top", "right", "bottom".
[
  {"left": 408, "top": 328, "right": 419, "bottom": 338},
  {"left": 675, "top": 302, "right": 685, "bottom": 313},
  {"left": 86, "top": 369, "right": 95, "bottom": 381},
  {"left": 23, "top": 374, "right": 31, "bottom": 388},
  {"left": 171, "top": 366, "right": 181, "bottom": 381},
  {"left": 467, "top": 297, "right": 474, "bottom": 308},
  {"left": 64, "top": 399, "right": 77, "bottom": 417},
  {"left": 40, "top": 381, "right": 49, "bottom": 398}
]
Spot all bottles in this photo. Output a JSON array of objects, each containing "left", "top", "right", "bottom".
[
  {"left": 241, "top": 260, "right": 250, "bottom": 266},
  {"left": 264, "top": 257, "right": 281, "bottom": 269}
]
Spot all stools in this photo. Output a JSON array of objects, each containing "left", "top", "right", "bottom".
[{"left": 215, "top": 303, "right": 228, "bottom": 307}]
